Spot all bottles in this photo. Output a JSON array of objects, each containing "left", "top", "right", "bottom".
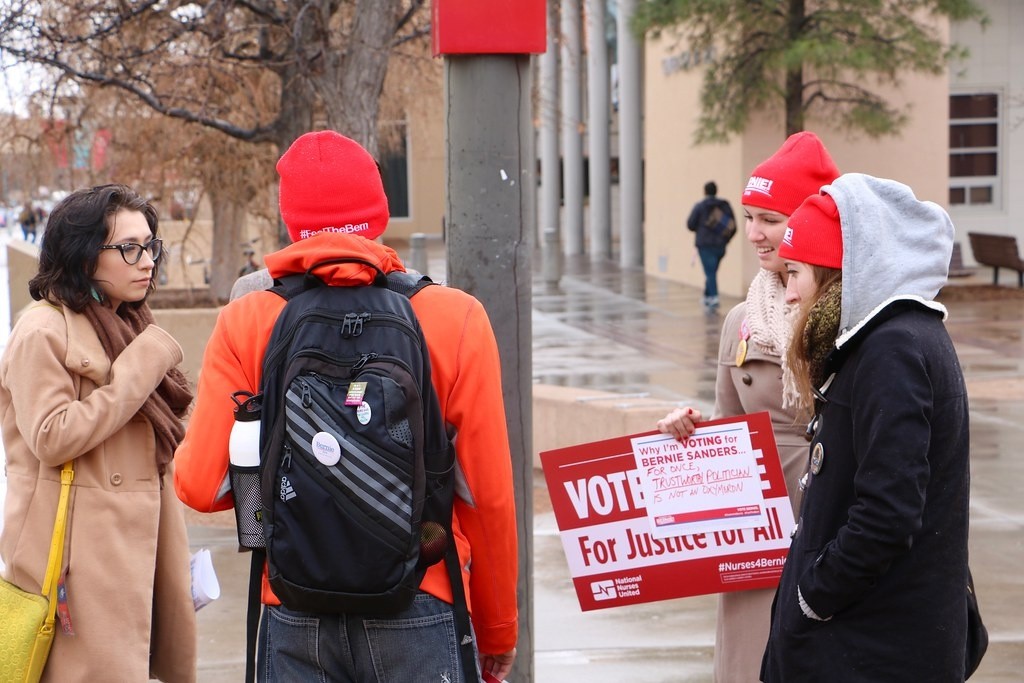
[{"left": 230, "top": 390, "right": 266, "bottom": 548}]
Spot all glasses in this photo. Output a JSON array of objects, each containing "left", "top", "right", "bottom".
[{"left": 97, "top": 238, "right": 163, "bottom": 265}]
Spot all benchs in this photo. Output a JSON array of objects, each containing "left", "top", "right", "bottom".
[
  {"left": 967, "top": 231, "right": 1024, "bottom": 289},
  {"left": 948, "top": 241, "right": 979, "bottom": 290}
]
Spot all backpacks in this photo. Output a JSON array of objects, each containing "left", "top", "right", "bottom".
[
  {"left": 705, "top": 200, "right": 737, "bottom": 239},
  {"left": 225, "top": 260, "right": 478, "bottom": 616}
]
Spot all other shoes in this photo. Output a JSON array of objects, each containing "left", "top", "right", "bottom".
[
  {"left": 711, "top": 297, "right": 720, "bottom": 305},
  {"left": 703, "top": 295, "right": 710, "bottom": 303}
]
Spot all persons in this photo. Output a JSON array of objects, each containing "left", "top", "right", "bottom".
[
  {"left": 18, "top": 199, "right": 37, "bottom": 243},
  {"left": 759, "top": 172, "right": 988, "bottom": 683},
  {"left": 657, "top": 130, "right": 843, "bottom": 683},
  {"left": 174, "top": 128, "right": 520, "bottom": 683},
  {"left": 0, "top": 184, "right": 195, "bottom": 683},
  {"left": 687, "top": 182, "right": 737, "bottom": 307}
]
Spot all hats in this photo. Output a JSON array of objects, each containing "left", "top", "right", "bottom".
[
  {"left": 778, "top": 192, "right": 843, "bottom": 268},
  {"left": 742, "top": 131, "right": 842, "bottom": 219},
  {"left": 278, "top": 130, "right": 390, "bottom": 243}
]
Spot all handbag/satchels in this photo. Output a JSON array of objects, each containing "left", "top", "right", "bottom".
[
  {"left": 0, "top": 577, "right": 56, "bottom": 683},
  {"left": 965, "top": 566, "right": 988, "bottom": 681}
]
